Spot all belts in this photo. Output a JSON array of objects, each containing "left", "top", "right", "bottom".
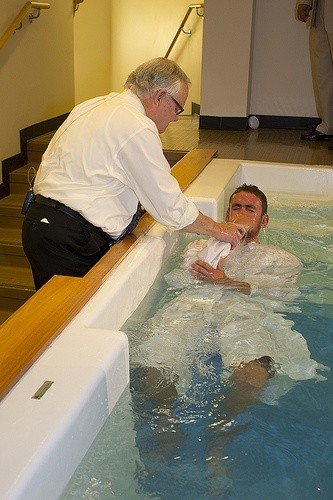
[{"left": 30, "top": 194, "right": 114, "bottom": 244}]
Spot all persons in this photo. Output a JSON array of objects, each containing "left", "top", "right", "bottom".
[
  {"left": 20, "top": 57, "right": 246, "bottom": 294},
  {"left": 294, "top": 0, "right": 333, "bottom": 152},
  {"left": 123, "top": 184, "right": 330, "bottom": 495}
]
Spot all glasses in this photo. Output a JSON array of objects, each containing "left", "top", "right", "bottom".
[{"left": 169, "top": 92, "right": 184, "bottom": 115}]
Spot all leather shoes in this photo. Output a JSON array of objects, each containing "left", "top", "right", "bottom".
[{"left": 300, "top": 130, "right": 333, "bottom": 150}]
